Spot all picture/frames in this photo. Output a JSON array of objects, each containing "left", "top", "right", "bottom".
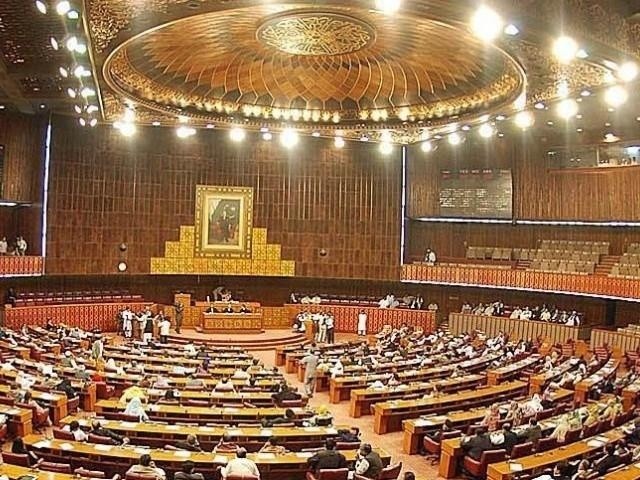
[{"left": 194, "top": 184, "right": 254, "bottom": 258}]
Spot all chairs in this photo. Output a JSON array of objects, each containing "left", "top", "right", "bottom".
[
  {"left": 10, "top": 288, "right": 145, "bottom": 307},
  {"left": 463, "top": 304, "right": 585, "bottom": 325},
  {"left": 413, "top": 238, "right": 640, "bottom": 281},
  {"left": 289, "top": 292, "right": 439, "bottom": 314}
]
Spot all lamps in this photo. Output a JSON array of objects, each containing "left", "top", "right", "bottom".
[{"left": 35, "top": 0, "right": 99, "bottom": 128}]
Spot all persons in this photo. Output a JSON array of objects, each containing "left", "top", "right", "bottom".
[
  {"left": 175, "top": 300, "right": 184, "bottom": 335},
  {"left": 206, "top": 303, "right": 251, "bottom": 314},
  {"left": 424, "top": 247, "right": 437, "bottom": 263},
  {"left": 116, "top": 306, "right": 171, "bottom": 345},
  {"left": 0, "top": 316, "right": 260, "bottom": 480},
  {"left": 216, "top": 210, "right": 229, "bottom": 242},
  {"left": 264, "top": 288, "right": 638, "bottom": 479},
  {"left": 0, "top": 235, "right": 27, "bottom": 256}
]
